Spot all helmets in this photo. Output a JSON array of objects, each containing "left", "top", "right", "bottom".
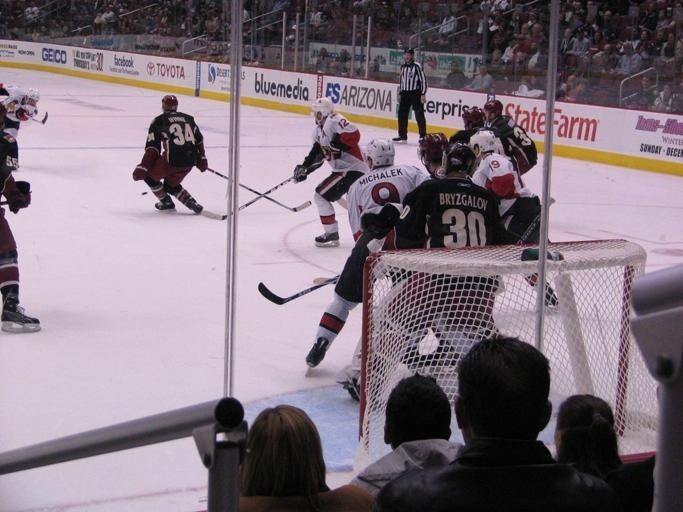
[
  {"left": 484, "top": 100, "right": 503, "bottom": 117},
  {"left": 365, "top": 138, "right": 395, "bottom": 166},
  {"left": 463, "top": 106, "right": 484, "bottom": 126},
  {"left": 162, "top": 95, "right": 178, "bottom": 111},
  {"left": 24, "top": 89, "right": 40, "bottom": 101},
  {"left": 442, "top": 144, "right": 476, "bottom": 177},
  {"left": 312, "top": 98, "right": 333, "bottom": 116},
  {"left": 469, "top": 128, "right": 497, "bottom": 153},
  {"left": 419, "top": 133, "right": 447, "bottom": 164}
]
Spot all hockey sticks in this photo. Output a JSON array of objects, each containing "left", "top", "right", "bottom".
[
  {"left": 199, "top": 175, "right": 293, "bottom": 220},
  {"left": 31, "top": 112, "right": 48, "bottom": 124},
  {"left": 208, "top": 168, "right": 311, "bottom": 211},
  {"left": 258, "top": 274, "right": 342, "bottom": 305}
]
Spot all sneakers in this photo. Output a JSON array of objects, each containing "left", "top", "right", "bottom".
[
  {"left": 2, "top": 302, "right": 40, "bottom": 326},
  {"left": 315, "top": 232, "right": 340, "bottom": 243},
  {"left": 392, "top": 136, "right": 407, "bottom": 142},
  {"left": 545, "top": 282, "right": 558, "bottom": 311},
  {"left": 155, "top": 198, "right": 175, "bottom": 210},
  {"left": 418, "top": 138, "right": 424, "bottom": 143},
  {"left": 347, "top": 373, "right": 360, "bottom": 400},
  {"left": 187, "top": 198, "right": 204, "bottom": 213},
  {"left": 305, "top": 343, "right": 326, "bottom": 368}
]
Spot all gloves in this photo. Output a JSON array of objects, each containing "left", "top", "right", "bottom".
[
  {"left": 197, "top": 157, "right": 209, "bottom": 172},
  {"left": 294, "top": 164, "right": 311, "bottom": 182},
  {"left": 329, "top": 143, "right": 342, "bottom": 159},
  {"left": 3, "top": 178, "right": 30, "bottom": 212},
  {"left": 17, "top": 109, "right": 32, "bottom": 120},
  {"left": 133, "top": 164, "right": 150, "bottom": 181}
]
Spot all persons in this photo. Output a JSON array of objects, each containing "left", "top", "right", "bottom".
[
  {"left": 242, "top": 0, "right": 551, "bottom": 98},
  {"left": 349, "top": 372, "right": 455, "bottom": 508},
  {"left": 606, "top": 454, "right": 658, "bottom": 512},
  {"left": 553, "top": 393, "right": 624, "bottom": 473},
  {"left": 556, "top": 0, "right": 683, "bottom": 115},
  {"left": 131, "top": 95, "right": 206, "bottom": 215},
  {"left": 239, "top": 402, "right": 371, "bottom": 510},
  {"left": 0, "top": 130, "right": 40, "bottom": 324},
  {"left": 0, "top": 86, "right": 39, "bottom": 140},
  {"left": 0, "top": 0, "right": 232, "bottom": 65},
  {"left": 291, "top": 92, "right": 563, "bottom": 405},
  {"left": 393, "top": 49, "right": 429, "bottom": 141},
  {"left": 369, "top": 339, "right": 618, "bottom": 512}
]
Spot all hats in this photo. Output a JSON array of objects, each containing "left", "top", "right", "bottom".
[{"left": 404, "top": 48, "right": 415, "bottom": 54}]
